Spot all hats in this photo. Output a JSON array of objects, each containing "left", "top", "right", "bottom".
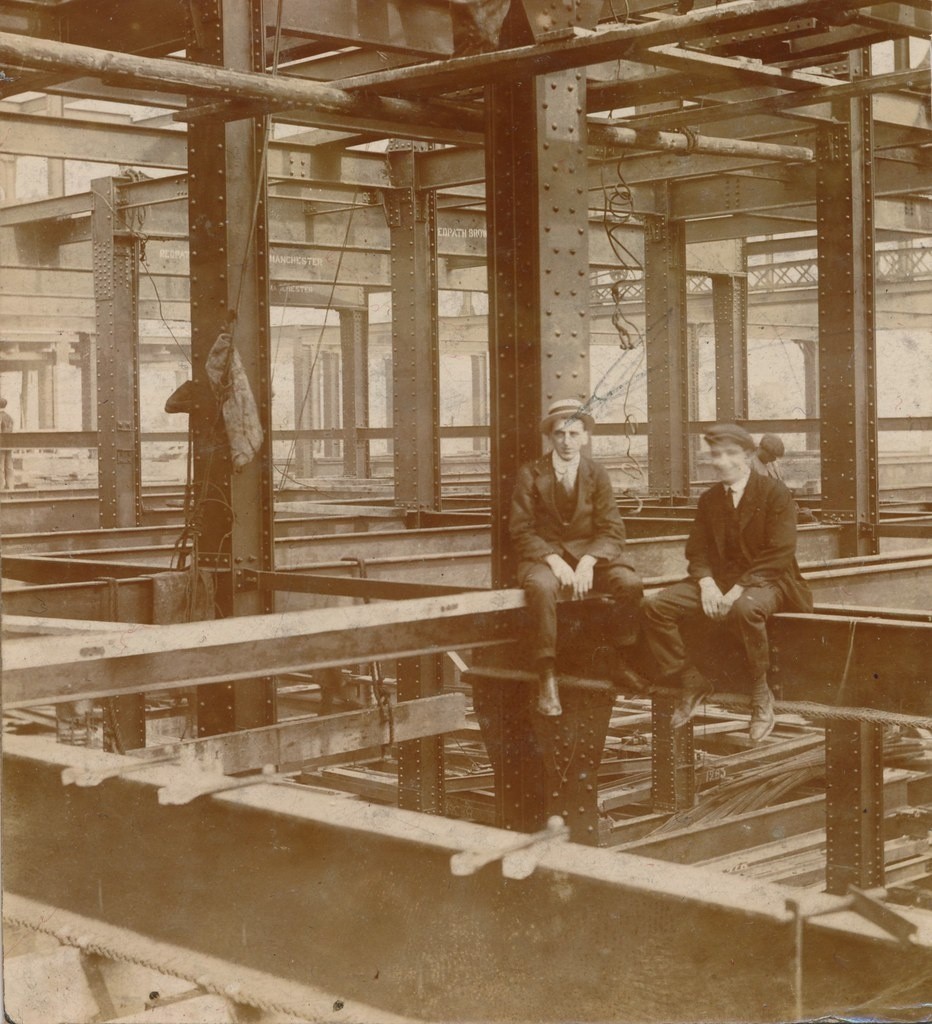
[
  {"left": 760, "top": 433, "right": 784, "bottom": 459},
  {"left": 704, "top": 425, "right": 755, "bottom": 452},
  {"left": 540, "top": 401, "right": 594, "bottom": 435}
]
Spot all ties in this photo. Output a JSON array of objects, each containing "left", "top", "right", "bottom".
[
  {"left": 765, "top": 463, "right": 777, "bottom": 480},
  {"left": 727, "top": 488, "right": 734, "bottom": 511},
  {"left": 561, "top": 465, "right": 572, "bottom": 494}
]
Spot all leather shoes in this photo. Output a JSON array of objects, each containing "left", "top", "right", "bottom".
[
  {"left": 670, "top": 677, "right": 713, "bottom": 728},
  {"left": 612, "top": 665, "right": 655, "bottom": 695},
  {"left": 537, "top": 665, "right": 562, "bottom": 717},
  {"left": 749, "top": 687, "right": 775, "bottom": 744}
]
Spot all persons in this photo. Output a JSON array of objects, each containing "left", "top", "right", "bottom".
[
  {"left": 505, "top": 400, "right": 657, "bottom": 720},
  {"left": 635, "top": 422, "right": 816, "bottom": 743},
  {"left": 0, "top": 397, "right": 16, "bottom": 490},
  {"left": 751, "top": 434, "right": 786, "bottom": 482}
]
